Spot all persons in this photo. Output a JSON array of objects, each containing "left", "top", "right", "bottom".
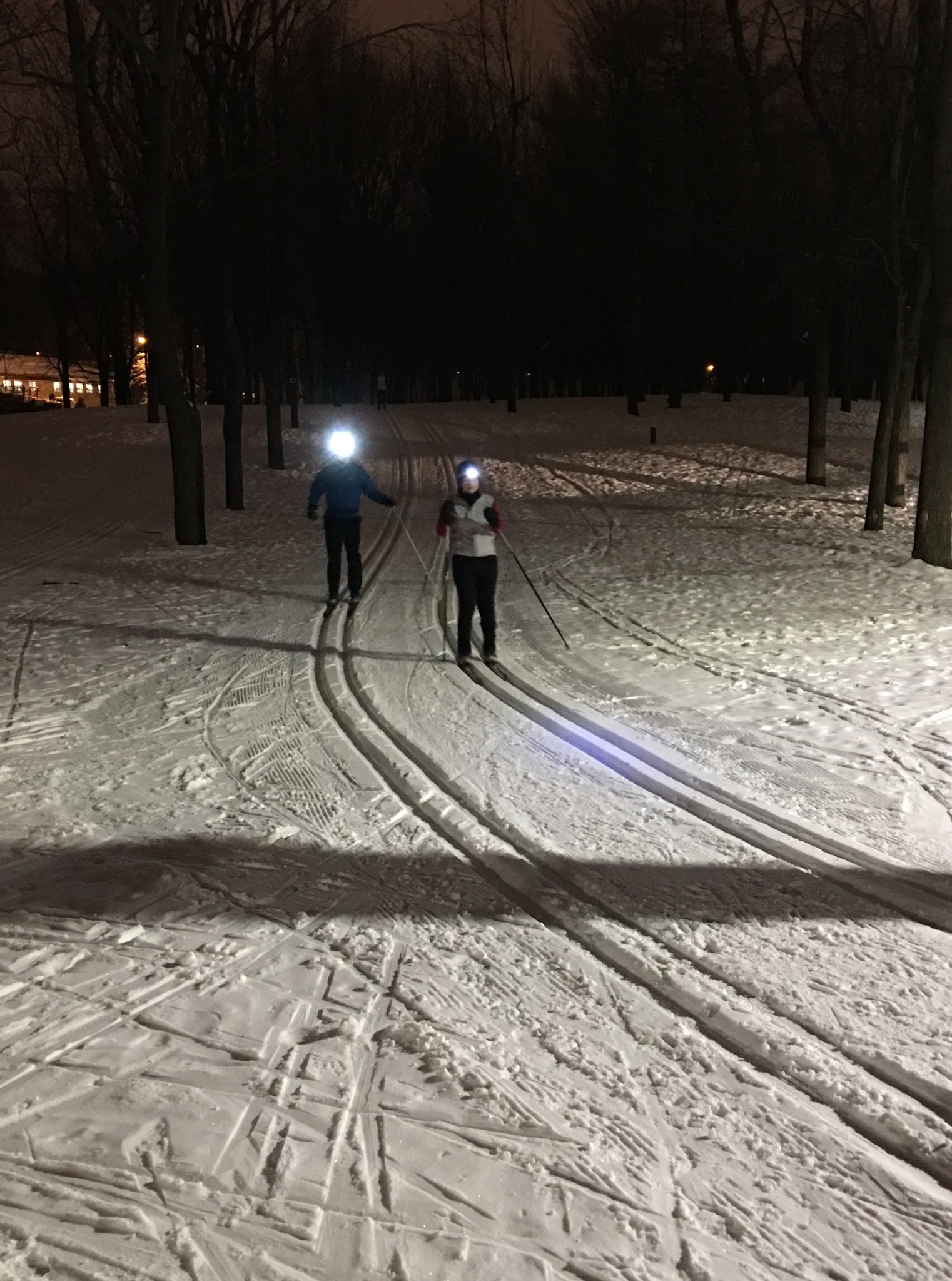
[
  {"left": 435, "top": 459, "right": 505, "bottom": 666},
  {"left": 377, "top": 370, "right": 388, "bottom": 410},
  {"left": 305, "top": 458, "right": 402, "bottom": 610}
]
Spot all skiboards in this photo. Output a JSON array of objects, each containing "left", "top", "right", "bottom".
[
  {"left": 322, "top": 591, "right": 362, "bottom": 619},
  {"left": 453, "top": 650, "right": 507, "bottom": 684}
]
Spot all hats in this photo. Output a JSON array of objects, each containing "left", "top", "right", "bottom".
[{"left": 456, "top": 460, "right": 480, "bottom": 479}]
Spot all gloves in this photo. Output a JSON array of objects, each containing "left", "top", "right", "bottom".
[
  {"left": 387, "top": 496, "right": 396, "bottom": 507},
  {"left": 484, "top": 506, "right": 498, "bottom": 525},
  {"left": 307, "top": 511, "right": 317, "bottom": 519},
  {"left": 436, "top": 523, "right": 447, "bottom": 536}
]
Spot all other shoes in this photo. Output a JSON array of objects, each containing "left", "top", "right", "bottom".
[
  {"left": 455, "top": 653, "right": 472, "bottom": 667},
  {"left": 481, "top": 654, "right": 499, "bottom": 665},
  {"left": 349, "top": 597, "right": 360, "bottom": 608},
  {"left": 327, "top": 599, "right": 337, "bottom": 611}
]
